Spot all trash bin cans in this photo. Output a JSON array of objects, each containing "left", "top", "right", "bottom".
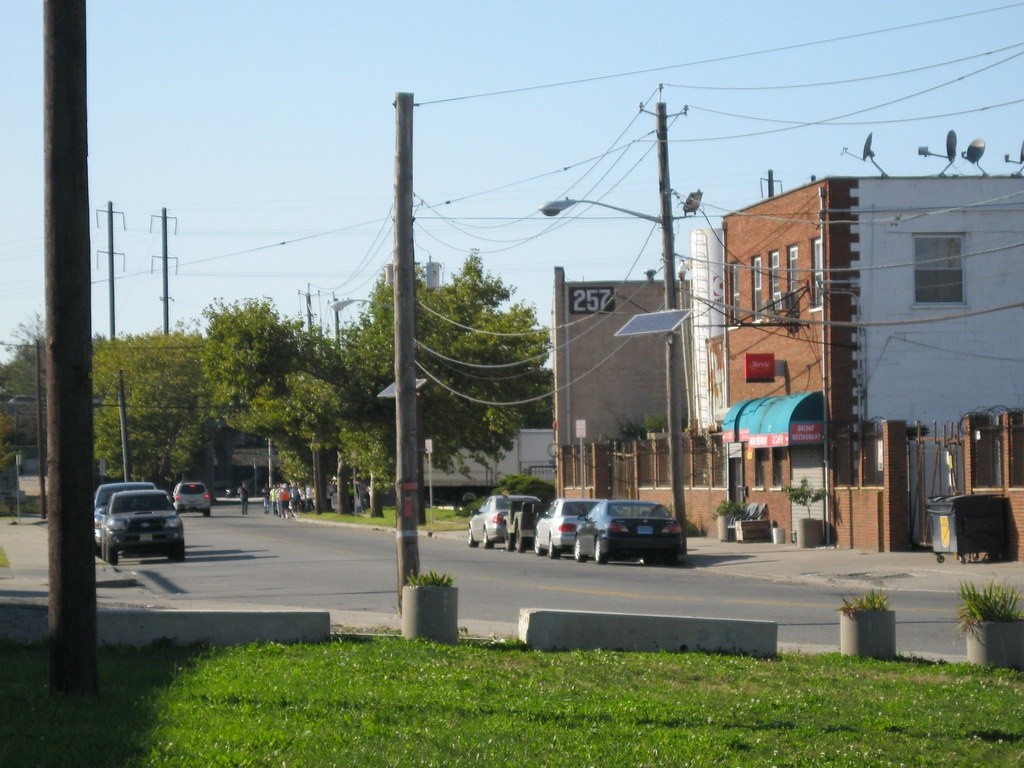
[{"left": 926, "top": 493, "right": 1007, "bottom": 564}]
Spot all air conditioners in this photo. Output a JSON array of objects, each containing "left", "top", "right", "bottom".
[{"left": 774, "top": 292, "right": 798, "bottom": 310}]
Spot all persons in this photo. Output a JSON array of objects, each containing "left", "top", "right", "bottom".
[
  {"left": 263, "top": 483, "right": 314, "bottom": 520},
  {"left": 239, "top": 482, "right": 250, "bottom": 515}
]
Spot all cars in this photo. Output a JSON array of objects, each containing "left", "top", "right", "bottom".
[
  {"left": 534, "top": 498, "right": 618, "bottom": 559},
  {"left": 467, "top": 495, "right": 542, "bottom": 548},
  {"left": 574, "top": 500, "right": 682, "bottom": 565}
]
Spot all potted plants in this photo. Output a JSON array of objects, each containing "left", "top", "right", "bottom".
[
  {"left": 401, "top": 569, "right": 459, "bottom": 642},
  {"left": 954, "top": 578, "right": 1024, "bottom": 672},
  {"left": 834, "top": 589, "right": 895, "bottom": 658},
  {"left": 713, "top": 500, "right": 745, "bottom": 541},
  {"left": 782, "top": 476, "right": 830, "bottom": 549}
]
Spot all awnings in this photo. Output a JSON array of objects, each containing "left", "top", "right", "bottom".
[
  {"left": 723, "top": 397, "right": 773, "bottom": 444},
  {"left": 749, "top": 393, "right": 821, "bottom": 448}
]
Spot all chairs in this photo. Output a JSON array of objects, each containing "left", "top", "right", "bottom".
[{"left": 727, "top": 501, "right": 767, "bottom": 542}]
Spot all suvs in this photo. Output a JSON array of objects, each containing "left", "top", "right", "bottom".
[
  {"left": 173, "top": 482, "right": 210, "bottom": 517},
  {"left": 500, "top": 489, "right": 552, "bottom": 553},
  {"left": 99, "top": 489, "right": 185, "bottom": 563},
  {"left": 95, "top": 482, "right": 157, "bottom": 552}
]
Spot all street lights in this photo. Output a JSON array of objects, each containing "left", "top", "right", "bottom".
[
  {"left": 539, "top": 197, "right": 687, "bottom": 532},
  {"left": 331, "top": 298, "right": 428, "bottom": 527}
]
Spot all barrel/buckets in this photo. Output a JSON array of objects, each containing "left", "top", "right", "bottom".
[{"left": 773, "top": 528, "right": 785, "bottom": 544}]
